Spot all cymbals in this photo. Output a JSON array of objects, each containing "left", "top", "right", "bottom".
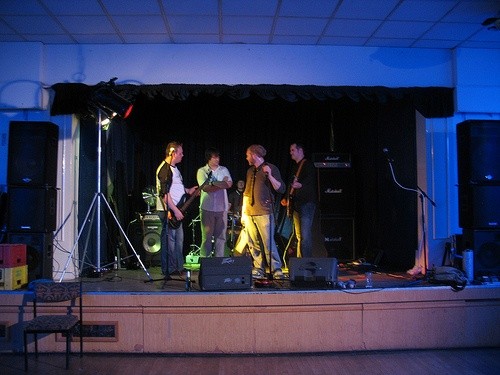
[{"left": 141, "top": 184, "right": 157, "bottom": 207}]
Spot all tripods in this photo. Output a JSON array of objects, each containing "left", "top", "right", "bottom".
[{"left": 252, "top": 174, "right": 288, "bottom": 290}]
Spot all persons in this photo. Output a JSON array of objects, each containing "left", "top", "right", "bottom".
[
  {"left": 155, "top": 142, "right": 201, "bottom": 277},
  {"left": 281, "top": 141, "right": 317, "bottom": 259},
  {"left": 197, "top": 150, "right": 233, "bottom": 257},
  {"left": 240, "top": 144, "right": 286, "bottom": 280},
  {"left": 227, "top": 179, "right": 245, "bottom": 213}
]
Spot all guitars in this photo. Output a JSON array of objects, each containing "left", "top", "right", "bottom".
[
  {"left": 285, "top": 173, "right": 298, "bottom": 219},
  {"left": 168, "top": 171, "right": 214, "bottom": 229}
]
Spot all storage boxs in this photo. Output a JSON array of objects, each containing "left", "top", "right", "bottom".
[
  {"left": 0, "top": 265, "right": 27, "bottom": 291},
  {"left": 0, "top": 243, "right": 27, "bottom": 267}
]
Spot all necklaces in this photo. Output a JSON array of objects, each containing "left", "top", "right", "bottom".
[{"left": 236, "top": 189, "right": 240, "bottom": 196}]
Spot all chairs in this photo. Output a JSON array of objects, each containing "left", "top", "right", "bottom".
[{"left": 21, "top": 281, "right": 84, "bottom": 372}]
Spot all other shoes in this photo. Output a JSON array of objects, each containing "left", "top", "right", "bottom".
[
  {"left": 273, "top": 268, "right": 284, "bottom": 279},
  {"left": 252, "top": 269, "right": 265, "bottom": 278},
  {"left": 170, "top": 268, "right": 191, "bottom": 276}
]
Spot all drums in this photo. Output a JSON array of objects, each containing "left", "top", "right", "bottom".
[{"left": 210, "top": 224, "right": 252, "bottom": 257}]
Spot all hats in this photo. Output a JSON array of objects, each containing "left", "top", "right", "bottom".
[{"left": 249, "top": 145, "right": 266, "bottom": 158}]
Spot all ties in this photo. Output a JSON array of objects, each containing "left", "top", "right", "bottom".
[{"left": 249, "top": 170, "right": 257, "bottom": 206}]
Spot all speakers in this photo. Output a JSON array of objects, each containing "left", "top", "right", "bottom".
[
  {"left": 131, "top": 221, "right": 163, "bottom": 266},
  {"left": 289, "top": 256, "right": 339, "bottom": 290},
  {"left": 316, "top": 166, "right": 356, "bottom": 216},
  {"left": 7, "top": 187, "right": 57, "bottom": 232},
  {"left": 198, "top": 256, "right": 253, "bottom": 291},
  {"left": 7, "top": 119, "right": 58, "bottom": 187},
  {"left": 457, "top": 119, "right": 500, "bottom": 278},
  {"left": 4, "top": 231, "right": 53, "bottom": 288},
  {"left": 313, "top": 216, "right": 355, "bottom": 262}
]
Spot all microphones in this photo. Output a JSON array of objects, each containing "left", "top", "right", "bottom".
[
  {"left": 382, "top": 148, "right": 391, "bottom": 162},
  {"left": 263, "top": 162, "right": 269, "bottom": 175},
  {"left": 168, "top": 147, "right": 176, "bottom": 156}
]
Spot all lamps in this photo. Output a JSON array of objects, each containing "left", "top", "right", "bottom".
[{"left": 58, "top": 77, "right": 155, "bottom": 284}]
